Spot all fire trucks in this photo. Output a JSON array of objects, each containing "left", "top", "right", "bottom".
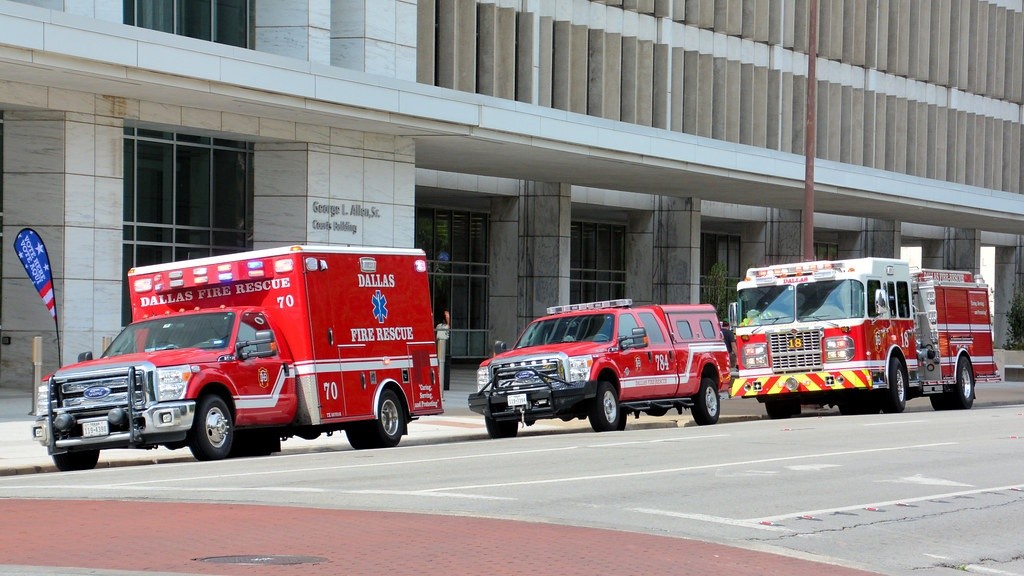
[
  {"left": 727, "top": 255, "right": 1003, "bottom": 419},
  {"left": 468, "top": 297, "right": 732, "bottom": 439}
]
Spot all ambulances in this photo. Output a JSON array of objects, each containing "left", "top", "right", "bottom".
[{"left": 32, "top": 244, "right": 446, "bottom": 472}]
